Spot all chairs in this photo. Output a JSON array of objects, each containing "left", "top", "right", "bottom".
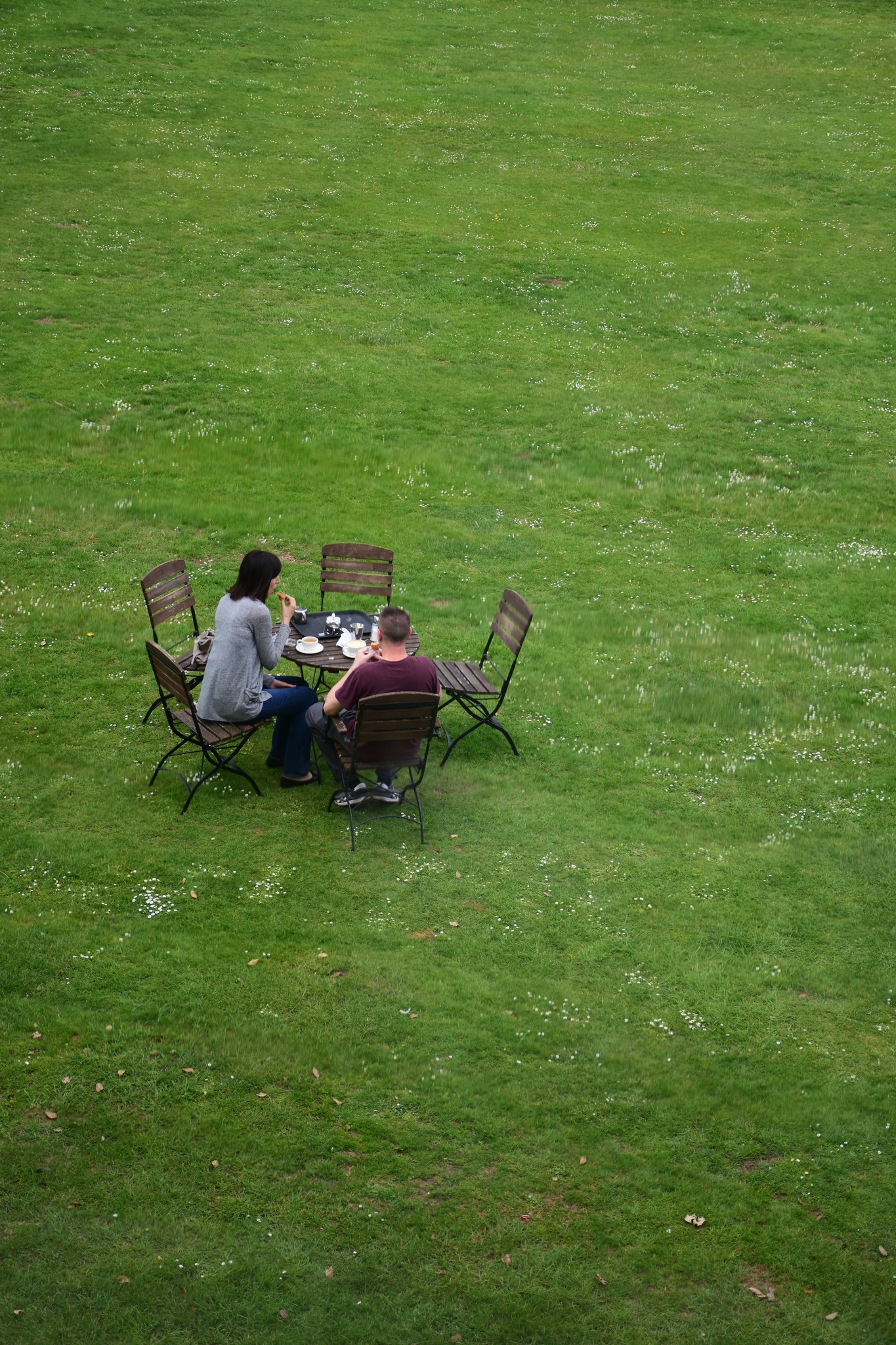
[
  {"left": 140, "top": 559, "right": 205, "bottom": 724},
  {"left": 319, "top": 543, "right": 394, "bottom": 612},
  {"left": 431, "top": 588, "right": 533, "bottom": 769},
  {"left": 144, "top": 640, "right": 274, "bottom": 815},
  {"left": 326, "top": 692, "right": 440, "bottom": 850}
]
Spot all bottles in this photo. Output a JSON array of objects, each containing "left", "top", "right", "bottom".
[{"left": 370, "top": 615, "right": 379, "bottom": 642}]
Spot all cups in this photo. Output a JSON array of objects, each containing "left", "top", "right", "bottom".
[
  {"left": 350, "top": 623, "right": 364, "bottom": 640},
  {"left": 342, "top": 640, "right": 367, "bottom": 656},
  {"left": 297, "top": 636, "right": 319, "bottom": 651}
]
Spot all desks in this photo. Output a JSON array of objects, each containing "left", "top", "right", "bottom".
[{"left": 271, "top": 613, "right": 420, "bottom": 786}]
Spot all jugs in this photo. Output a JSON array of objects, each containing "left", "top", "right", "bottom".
[
  {"left": 294, "top": 606, "right": 307, "bottom": 625},
  {"left": 325, "top": 612, "right": 342, "bottom": 635}
]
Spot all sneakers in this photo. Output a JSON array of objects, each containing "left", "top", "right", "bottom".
[
  {"left": 333, "top": 782, "right": 371, "bottom": 806},
  {"left": 373, "top": 783, "right": 399, "bottom": 802}
]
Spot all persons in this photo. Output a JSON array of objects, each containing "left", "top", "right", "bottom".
[
  {"left": 306, "top": 605, "right": 440, "bottom": 804},
  {"left": 194, "top": 550, "right": 321, "bottom": 787}
]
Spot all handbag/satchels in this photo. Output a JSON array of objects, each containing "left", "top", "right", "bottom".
[{"left": 191, "top": 627, "right": 215, "bottom": 665}]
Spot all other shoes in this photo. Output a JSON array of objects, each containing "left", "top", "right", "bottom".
[
  {"left": 280, "top": 770, "right": 319, "bottom": 788},
  {"left": 266, "top": 755, "right": 314, "bottom": 768}
]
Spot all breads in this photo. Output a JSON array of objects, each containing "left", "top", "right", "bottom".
[
  {"left": 371, "top": 642, "right": 379, "bottom": 650},
  {"left": 278, "top": 590, "right": 286, "bottom": 602}
]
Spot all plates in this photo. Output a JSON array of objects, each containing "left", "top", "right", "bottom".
[
  {"left": 296, "top": 642, "right": 323, "bottom": 654},
  {"left": 343, "top": 648, "right": 356, "bottom": 658}
]
